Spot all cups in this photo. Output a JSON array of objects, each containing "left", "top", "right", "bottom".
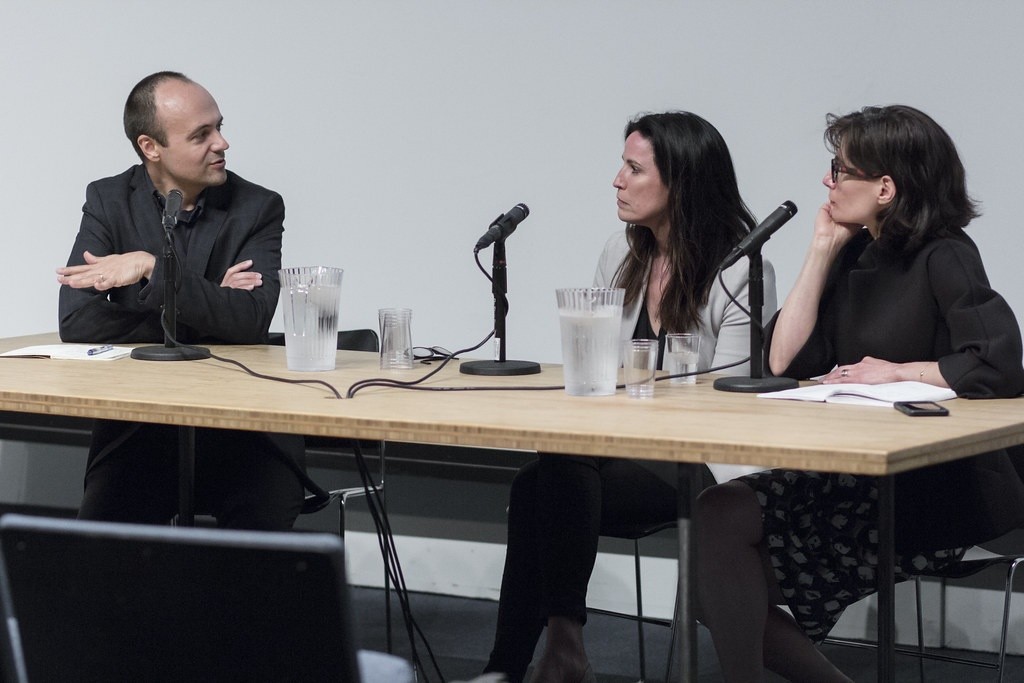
[
  {"left": 623, "top": 332, "right": 701, "bottom": 396},
  {"left": 277, "top": 264, "right": 344, "bottom": 373},
  {"left": 555, "top": 286, "right": 627, "bottom": 396},
  {"left": 378, "top": 308, "right": 414, "bottom": 371}
]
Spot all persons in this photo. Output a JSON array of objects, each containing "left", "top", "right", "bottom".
[
  {"left": 689, "top": 103, "right": 1024, "bottom": 683},
  {"left": 56, "top": 69, "right": 302, "bottom": 534},
  {"left": 479, "top": 112, "right": 777, "bottom": 683}
]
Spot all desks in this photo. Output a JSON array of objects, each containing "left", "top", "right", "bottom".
[{"left": 0, "top": 331, "right": 1024, "bottom": 683}]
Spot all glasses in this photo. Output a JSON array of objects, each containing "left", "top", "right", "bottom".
[
  {"left": 405, "top": 346, "right": 459, "bottom": 364},
  {"left": 830, "top": 158, "right": 877, "bottom": 183}
]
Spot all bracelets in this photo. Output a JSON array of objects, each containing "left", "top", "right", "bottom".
[{"left": 920, "top": 361, "right": 931, "bottom": 382}]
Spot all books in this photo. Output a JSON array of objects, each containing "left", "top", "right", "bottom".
[
  {"left": 758, "top": 381, "right": 957, "bottom": 408},
  {"left": 0, "top": 344, "right": 134, "bottom": 361}
]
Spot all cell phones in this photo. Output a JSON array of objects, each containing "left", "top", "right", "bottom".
[{"left": 894, "top": 401, "right": 948, "bottom": 417}]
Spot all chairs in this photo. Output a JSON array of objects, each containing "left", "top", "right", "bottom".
[
  {"left": 286, "top": 327, "right": 388, "bottom": 536},
  {"left": 592, "top": 522, "right": 1024, "bottom": 682},
  {"left": 0, "top": 513, "right": 363, "bottom": 682}
]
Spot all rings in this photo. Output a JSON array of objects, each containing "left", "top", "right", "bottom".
[
  {"left": 100, "top": 275, "right": 103, "bottom": 279},
  {"left": 841, "top": 370, "right": 848, "bottom": 377}
]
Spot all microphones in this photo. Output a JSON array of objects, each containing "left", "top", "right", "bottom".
[
  {"left": 477, "top": 203, "right": 530, "bottom": 250},
  {"left": 719, "top": 200, "right": 798, "bottom": 270},
  {"left": 161, "top": 189, "right": 183, "bottom": 233}
]
